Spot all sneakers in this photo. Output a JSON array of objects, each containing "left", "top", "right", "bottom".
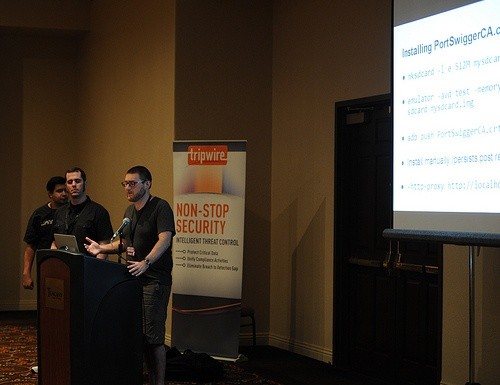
[{"left": 31, "top": 366, "right": 38, "bottom": 373}]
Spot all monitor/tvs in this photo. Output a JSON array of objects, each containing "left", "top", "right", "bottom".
[{"left": 54, "top": 233, "right": 79, "bottom": 254}]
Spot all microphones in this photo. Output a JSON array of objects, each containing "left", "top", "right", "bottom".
[{"left": 111, "top": 217, "right": 131, "bottom": 241}]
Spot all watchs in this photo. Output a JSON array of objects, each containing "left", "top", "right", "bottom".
[{"left": 143, "top": 258, "right": 151, "bottom": 265}]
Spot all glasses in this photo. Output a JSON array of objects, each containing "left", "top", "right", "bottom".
[{"left": 121, "top": 181, "right": 144, "bottom": 187}]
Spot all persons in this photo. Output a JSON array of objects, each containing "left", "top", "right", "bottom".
[
  {"left": 23, "top": 176, "right": 70, "bottom": 372},
  {"left": 83, "top": 165, "right": 177, "bottom": 385},
  {"left": 52, "top": 168, "right": 114, "bottom": 260}
]
